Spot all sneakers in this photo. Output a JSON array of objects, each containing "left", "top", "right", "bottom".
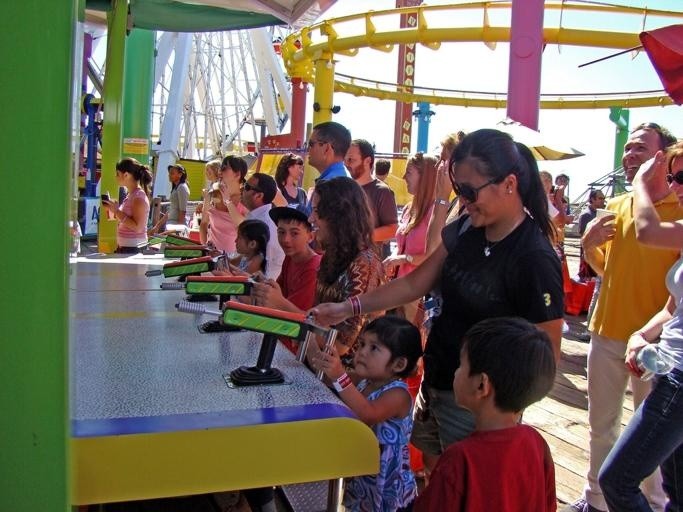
[{"left": 559, "top": 498, "right": 608, "bottom": 512}]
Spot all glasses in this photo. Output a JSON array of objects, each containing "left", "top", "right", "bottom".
[
  {"left": 598, "top": 197, "right": 605, "bottom": 200},
  {"left": 219, "top": 165, "right": 231, "bottom": 171},
  {"left": 666, "top": 170, "right": 683, "bottom": 185},
  {"left": 244, "top": 183, "right": 262, "bottom": 192},
  {"left": 451, "top": 181, "right": 492, "bottom": 203},
  {"left": 309, "top": 141, "right": 336, "bottom": 152},
  {"left": 630, "top": 122, "right": 661, "bottom": 135},
  {"left": 415, "top": 150, "right": 424, "bottom": 168}
]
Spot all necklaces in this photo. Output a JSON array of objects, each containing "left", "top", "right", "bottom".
[{"left": 484, "top": 210, "right": 525, "bottom": 257}]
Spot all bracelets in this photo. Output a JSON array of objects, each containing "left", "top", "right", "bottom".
[
  {"left": 120, "top": 215, "right": 128, "bottom": 226},
  {"left": 627, "top": 331, "right": 646, "bottom": 341},
  {"left": 332, "top": 371, "right": 353, "bottom": 393},
  {"left": 347, "top": 296, "right": 364, "bottom": 318}
]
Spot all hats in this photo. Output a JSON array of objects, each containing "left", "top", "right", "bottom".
[{"left": 268, "top": 203, "right": 313, "bottom": 232}]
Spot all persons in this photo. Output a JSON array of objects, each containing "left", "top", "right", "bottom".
[
  {"left": 249, "top": 176, "right": 387, "bottom": 397},
  {"left": 563, "top": 123, "right": 683, "bottom": 512},
  {"left": 598, "top": 140, "right": 683, "bottom": 511},
  {"left": 306, "top": 128, "right": 564, "bottom": 483},
  {"left": 101, "top": 157, "right": 151, "bottom": 254},
  {"left": 297, "top": 122, "right": 466, "bottom": 398},
  {"left": 146, "top": 164, "right": 190, "bottom": 237},
  {"left": 539, "top": 171, "right": 606, "bottom": 342},
  {"left": 286, "top": 317, "right": 426, "bottom": 511},
  {"left": 200, "top": 152, "right": 320, "bottom": 355}
]
